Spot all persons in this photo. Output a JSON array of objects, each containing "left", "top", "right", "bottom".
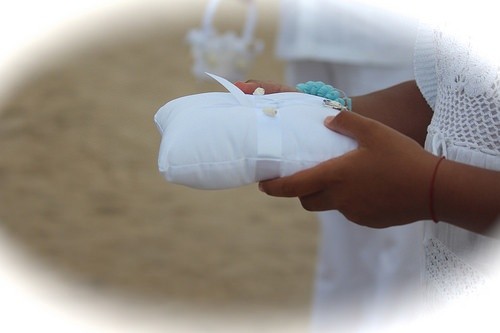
[{"left": 230, "top": 16, "right": 500, "bottom": 309}]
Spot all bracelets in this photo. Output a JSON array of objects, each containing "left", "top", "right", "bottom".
[
  {"left": 297, "top": 80, "right": 352, "bottom": 111},
  {"left": 428, "top": 154, "right": 447, "bottom": 224}
]
